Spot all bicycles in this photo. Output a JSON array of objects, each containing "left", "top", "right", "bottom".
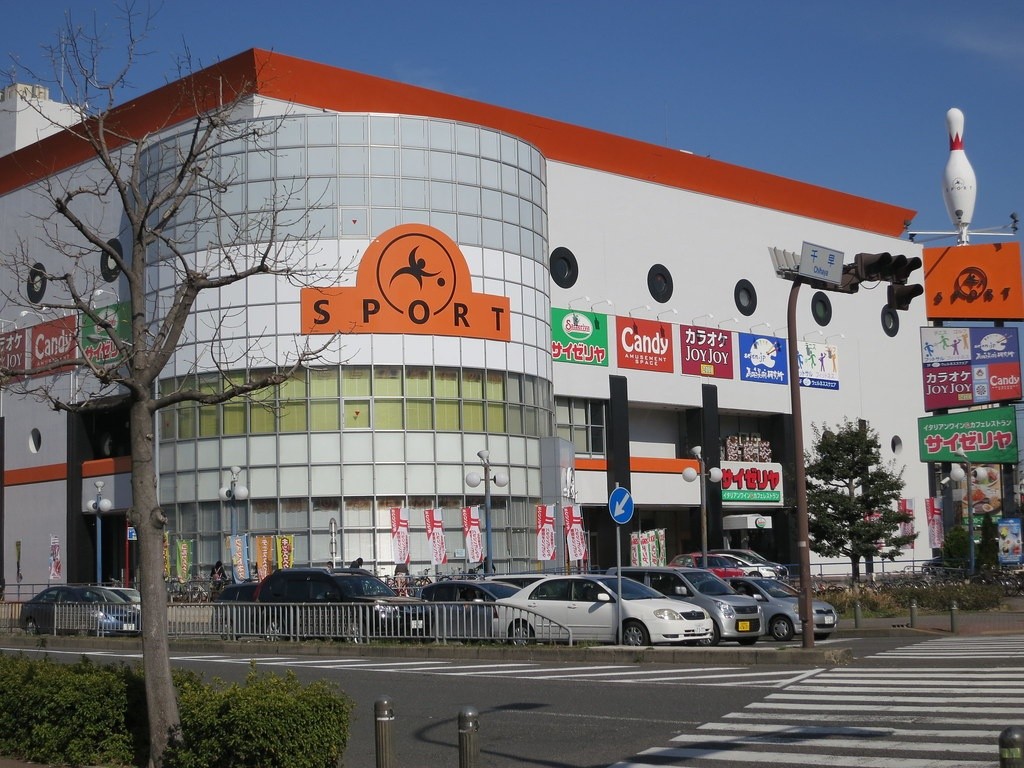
[
  {"left": 368, "top": 567, "right": 476, "bottom": 597},
  {"left": 858, "top": 564, "right": 1024, "bottom": 597}
]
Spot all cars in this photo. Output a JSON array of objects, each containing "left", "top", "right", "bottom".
[
  {"left": 415, "top": 574, "right": 553, "bottom": 644},
  {"left": 20, "top": 585, "right": 141, "bottom": 637},
  {"left": 605, "top": 566, "right": 765, "bottom": 647},
  {"left": 708, "top": 550, "right": 790, "bottom": 580},
  {"left": 921, "top": 556, "right": 977, "bottom": 575},
  {"left": 250, "top": 568, "right": 436, "bottom": 646},
  {"left": 211, "top": 582, "right": 261, "bottom": 640},
  {"left": 695, "top": 577, "right": 837, "bottom": 642},
  {"left": 491, "top": 574, "right": 713, "bottom": 646},
  {"left": 716, "top": 554, "right": 778, "bottom": 580},
  {"left": 667, "top": 554, "right": 748, "bottom": 579}
]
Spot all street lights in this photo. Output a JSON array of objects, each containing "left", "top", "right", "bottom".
[
  {"left": 87, "top": 481, "right": 112, "bottom": 586},
  {"left": 950, "top": 467, "right": 987, "bottom": 575},
  {"left": 218, "top": 466, "right": 248, "bottom": 584},
  {"left": 682, "top": 446, "right": 723, "bottom": 569},
  {"left": 466, "top": 450, "right": 509, "bottom": 576}
]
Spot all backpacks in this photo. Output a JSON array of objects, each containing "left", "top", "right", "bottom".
[{"left": 213, "top": 567, "right": 222, "bottom": 581}]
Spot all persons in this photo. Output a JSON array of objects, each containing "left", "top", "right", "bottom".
[
  {"left": 326, "top": 561, "right": 333, "bottom": 568},
  {"left": 476, "top": 557, "right": 496, "bottom": 574},
  {"left": 209, "top": 561, "right": 227, "bottom": 601},
  {"left": 350, "top": 558, "right": 363, "bottom": 568}
]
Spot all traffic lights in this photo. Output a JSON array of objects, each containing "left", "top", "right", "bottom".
[{"left": 853, "top": 253, "right": 924, "bottom": 310}]
